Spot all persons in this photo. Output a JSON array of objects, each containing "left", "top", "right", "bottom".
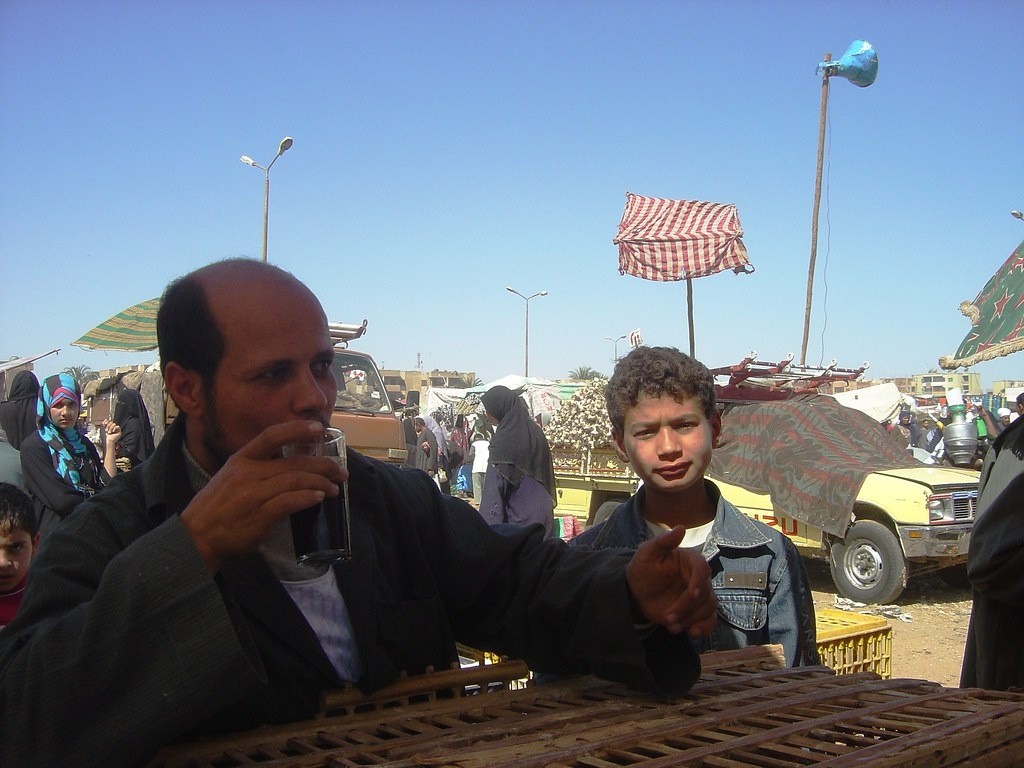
[
  {"left": 960, "top": 414, "right": 1024, "bottom": 693},
  {"left": 0, "top": 368, "right": 40, "bottom": 450},
  {"left": 533, "top": 347, "right": 820, "bottom": 691},
  {"left": 0, "top": 423, "right": 29, "bottom": 498},
  {"left": 100, "top": 389, "right": 155, "bottom": 474},
  {"left": 20, "top": 375, "right": 121, "bottom": 555},
  {"left": 0, "top": 260, "right": 718, "bottom": 768},
  {"left": 402, "top": 410, "right": 490, "bottom": 506},
  {"left": 478, "top": 386, "right": 557, "bottom": 543},
  {"left": 880, "top": 392, "right": 1024, "bottom": 473},
  {"left": 0, "top": 483, "right": 41, "bottom": 632}
]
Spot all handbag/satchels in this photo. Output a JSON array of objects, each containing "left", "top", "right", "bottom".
[{"left": 444, "top": 439, "right": 464, "bottom": 467}]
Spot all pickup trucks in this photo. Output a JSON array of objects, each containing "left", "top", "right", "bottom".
[{"left": 541, "top": 351, "right": 982, "bottom": 606}]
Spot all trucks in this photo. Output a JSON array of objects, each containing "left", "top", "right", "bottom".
[{"left": 85, "top": 319, "right": 409, "bottom": 470}]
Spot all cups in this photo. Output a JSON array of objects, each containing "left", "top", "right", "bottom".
[{"left": 279, "top": 427, "right": 353, "bottom": 568}]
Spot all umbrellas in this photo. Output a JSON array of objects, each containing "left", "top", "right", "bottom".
[
  {"left": 69, "top": 297, "right": 162, "bottom": 351},
  {"left": 939, "top": 238, "right": 1024, "bottom": 371}
]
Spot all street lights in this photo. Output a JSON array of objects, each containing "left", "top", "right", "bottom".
[
  {"left": 605, "top": 335, "right": 627, "bottom": 365},
  {"left": 240, "top": 136, "right": 295, "bottom": 265},
  {"left": 505, "top": 286, "right": 549, "bottom": 377}
]
